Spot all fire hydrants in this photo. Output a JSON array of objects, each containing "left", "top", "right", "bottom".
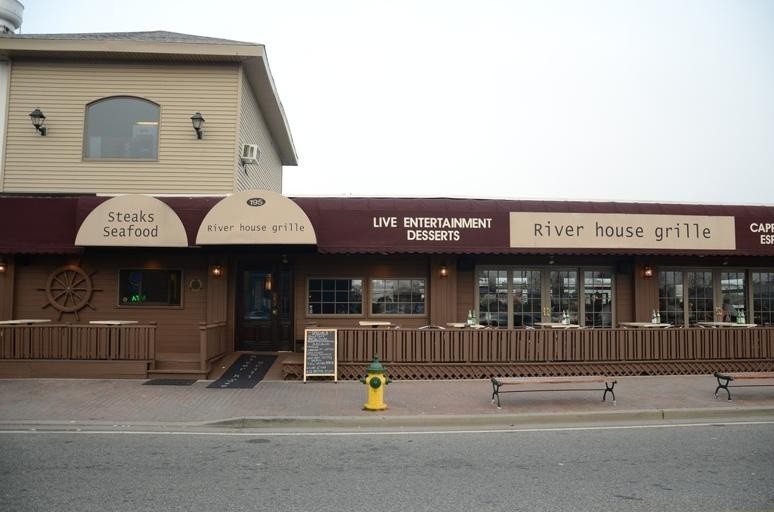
[{"left": 357, "top": 348, "right": 393, "bottom": 412}]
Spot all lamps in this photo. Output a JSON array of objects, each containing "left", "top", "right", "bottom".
[
  {"left": 644, "top": 266, "right": 653, "bottom": 280},
  {"left": 190, "top": 112, "right": 205, "bottom": 138},
  {"left": 29, "top": 107, "right": 47, "bottom": 136},
  {"left": 212, "top": 264, "right": 222, "bottom": 278},
  {"left": 440, "top": 262, "right": 449, "bottom": 279}
]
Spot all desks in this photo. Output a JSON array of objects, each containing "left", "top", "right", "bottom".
[
  {"left": 698, "top": 322, "right": 757, "bottom": 330},
  {"left": 447, "top": 323, "right": 485, "bottom": 329},
  {"left": 618, "top": 321, "right": 671, "bottom": 329},
  {"left": 535, "top": 323, "right": 579, "bottom": 328},
  {"left": 359, "top": 320, "right": 391, "bottom": 328}
]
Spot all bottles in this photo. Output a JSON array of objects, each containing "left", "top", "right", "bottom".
[
  {"left": 736, "top": 308, "right": 745, "bottom": 324},
  {"left": 309, "top": 296, "right": 313, "bottom": 314},
  {"left": 651, "top": 309, "right": 661, "bottom": 324},
  {"left": 562, "top": 309, "right": 570, "bottom": 325},
  {"left": 467, "top": 308, "right": 476, "bottom": 325}
]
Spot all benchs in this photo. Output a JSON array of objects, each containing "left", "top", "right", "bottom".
[
  {"left": 714, "top": 372, "right": 774, "bottom": 402},
  {"left": 491, "top": 376, "right": 617, "bottom": 410}
]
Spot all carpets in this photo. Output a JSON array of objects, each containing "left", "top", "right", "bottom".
[
  {"left": 206, "top": 353, "right": 281, "bottom": 389},
  {"left": 142, "top": 378, "right": 196, "bottom": 386}
]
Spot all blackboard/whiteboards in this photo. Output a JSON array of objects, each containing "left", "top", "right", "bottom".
[{"left": 304, "top": 329, "right": 337, "bottom": 377}]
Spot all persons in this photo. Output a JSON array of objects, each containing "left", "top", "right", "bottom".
[
  {"left": 480, "top": 284, "right": 611, "bottom": 328},
  {"left": 338, "top": 288, "right": 425, "bottom": 314},
  {"left": 659, "top": 288, "right": 737, "bottom": 324}
]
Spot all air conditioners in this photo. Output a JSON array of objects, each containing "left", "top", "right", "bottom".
[{"left": 240, "top": 144, "right": 261, "bottom": 165}]
